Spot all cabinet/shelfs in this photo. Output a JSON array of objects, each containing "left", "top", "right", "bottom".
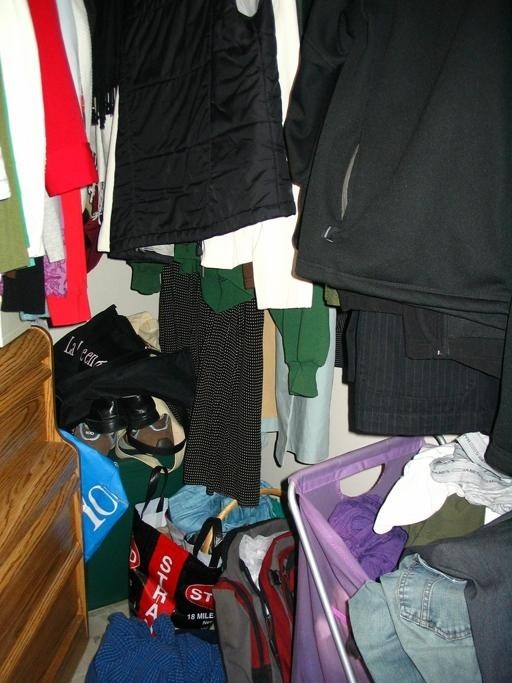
[{"left": 1, "top": 324, "right": 88, "bottom": 683}]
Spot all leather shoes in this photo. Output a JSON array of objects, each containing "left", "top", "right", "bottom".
[
  {"left": 121, "top": 394, "right": 159, "bottom": 429},
  {"left": 85, "top": 399, "right": 119, "bottom": 433}
]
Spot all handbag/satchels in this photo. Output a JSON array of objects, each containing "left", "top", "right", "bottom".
[
  {"left": 53, "top": 305, "right": 194, "bottom": 429},
  {"left": 128, "top": 466, "right": 222, "bottom": 637}
]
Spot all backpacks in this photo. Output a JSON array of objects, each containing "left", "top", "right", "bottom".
[{"left": 212, "top": 517, "right": 297, "bottom": 683}]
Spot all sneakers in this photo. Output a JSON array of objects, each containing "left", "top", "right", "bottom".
[
  {"left": 70, "top": 423, "right": 115, "bottom": 458},
  {"left": 117, "top": 414, "right": 174, "bottom": 450}
]
